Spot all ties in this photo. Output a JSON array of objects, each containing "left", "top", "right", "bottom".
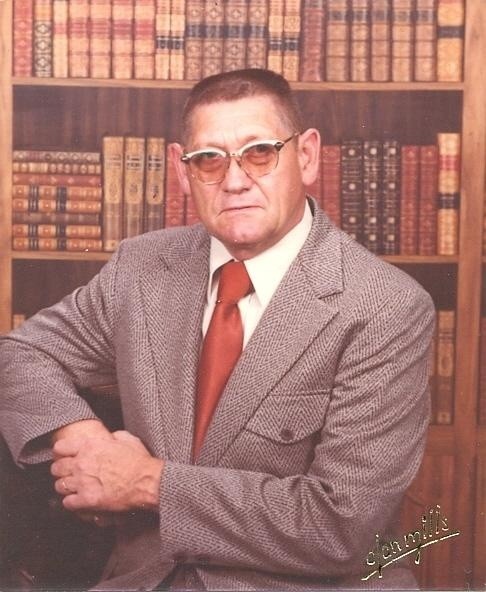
[{"left": 192, "top": 258, "right": 254, "bottom": 466}]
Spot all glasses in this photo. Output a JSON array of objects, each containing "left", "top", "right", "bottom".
[{"left": 181, "top": 130, "right": 301, "bottom": 185}]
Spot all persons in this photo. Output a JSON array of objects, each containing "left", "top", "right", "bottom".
[{"left": 0, "top": 68, "right": 437, "bottom": 592}]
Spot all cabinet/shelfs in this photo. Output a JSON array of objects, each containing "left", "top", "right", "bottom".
[{"left": 0, "top": 0, "right": 486, "bottom": 591}]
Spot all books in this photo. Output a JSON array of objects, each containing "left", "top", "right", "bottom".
[
  {"left": 436, "top": 311, "right": 454, "bottom": 427},
  {"left": 11, "top": 0, "right": 464, "bottom": 81},
  {"left": 303, "top": 132, "right": 459, "bottom": 258},
  {"left": 11, "top": 136, "right": 202, "bottom": 251}
]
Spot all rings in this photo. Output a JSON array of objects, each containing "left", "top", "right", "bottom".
[{"left": 60, "top": 477, "right": 73, "bottom": 495}]
